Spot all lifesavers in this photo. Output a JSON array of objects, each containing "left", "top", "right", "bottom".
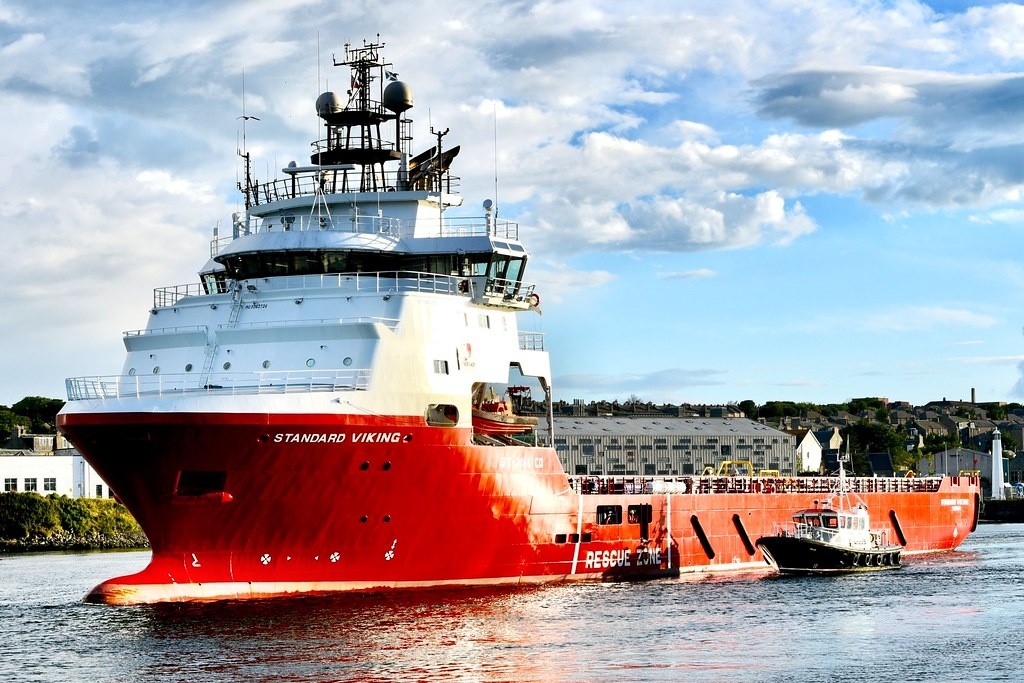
[
  {"left": 849, "top": 551, "right": 903, "bottom": 567},
  {"left": 531, "top": 293, "right": 540, "bottom": 307}
]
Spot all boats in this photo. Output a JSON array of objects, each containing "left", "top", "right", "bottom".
[
  {"left": 54, "top": 31, "right": 984, "bottom": 606},
  {"left": 754, "top": 453, "right": 905, "bottom": 577}
]
